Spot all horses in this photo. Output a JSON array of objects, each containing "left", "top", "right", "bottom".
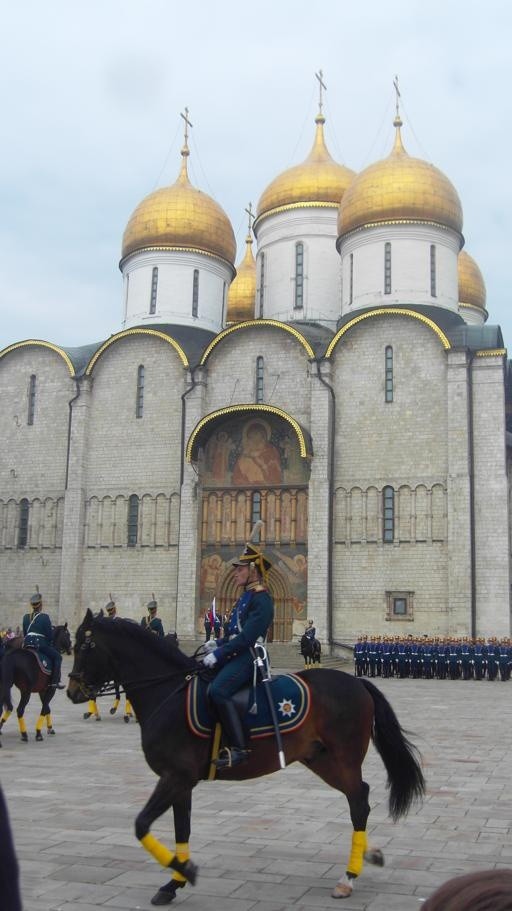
[
  {"left": 67, "top": 607, "right": 426, "bottom": 905},
  {"left": 84, "top": 631, "right": 180, "bottom": 723},
  {"left": 300, "top": 634, "right": 321, "bottom": 670},
  {"left": 0, "top": 623, "right": 73, "bottom": 742}
]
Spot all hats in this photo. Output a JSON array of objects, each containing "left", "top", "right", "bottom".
[
  {"left": 231, "top": 542, "right": 273, "bottom": 578},
  {"left": 206, "top": 608, "right": 230, "bottom": 615},
  {"left": 29, "top": 593, "right": 43, "bottom": 608},
  {"left": 357, "top": 634, "right": 511, "bottom": 644},
  {"left": 146, "top": 600, "right": 157, "bottom": 613},
  {"left": 308, "top": 620, "right": 313, "bottom": 624},
  {"left": 105, "top": 601, "right": 116, "bottom": 613}
]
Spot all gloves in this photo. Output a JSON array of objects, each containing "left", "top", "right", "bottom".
[
  {"left": 354, "top": 657, "right": 512, "bottom": 665},
  {"left": 203, "top": 640, "right": 218, "bottom": 669},
  {"left": 306, "top": 636, "right": 311, "bottom": 639}
]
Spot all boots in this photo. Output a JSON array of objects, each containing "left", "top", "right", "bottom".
[
  {"left": 356, "top": 671, "right": 512, "bottom": 681},
  {"left": 213, "top": 701, "right": 252, "bottom": 767}
]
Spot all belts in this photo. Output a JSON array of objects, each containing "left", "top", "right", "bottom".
[
  {"left": 25, "top": 629, "right": 46, "bottom": 640},
  {"left": 225, "top": 633, "right": 264, "bottom": 648},
  {"left": 355, "top": 649, "right": 508, "bottom": 657}
]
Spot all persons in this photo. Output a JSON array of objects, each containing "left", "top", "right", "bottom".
[
  {"left": 299, "top": 619, "right": 316, "bottom": 654},
  {"left": 353, "top": 634, "right": 512, "bottom": 682},
  {"left": 202, "top": 540, "right": 275, "bottom": 770},
  {"left": 0, "top": 594, "right": 166, "bottom": 689},
  {"left": 420, "top": 868, "right": 512, "bottom": 911}
]
